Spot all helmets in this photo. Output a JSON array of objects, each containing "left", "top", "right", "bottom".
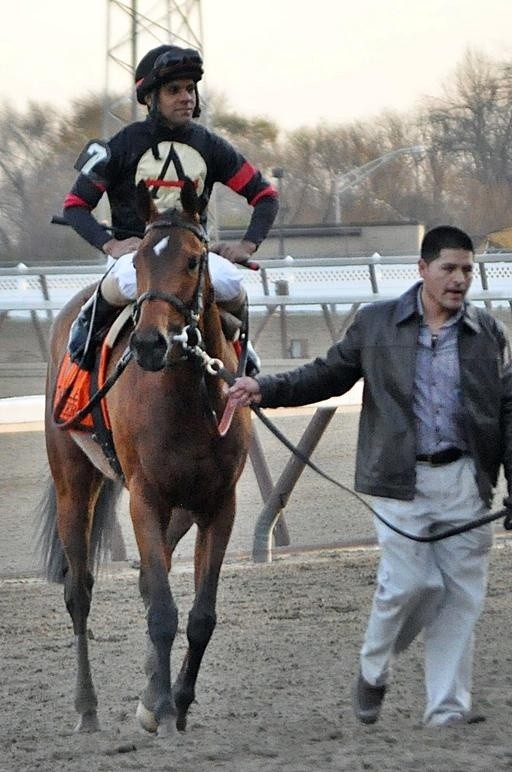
[{"left": 135, "top": 45, "right": 203, "bottom": 105}]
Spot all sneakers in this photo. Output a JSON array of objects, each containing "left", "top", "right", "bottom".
[{"left": 358, "top": 675, "right": 385, "bottom": 724}]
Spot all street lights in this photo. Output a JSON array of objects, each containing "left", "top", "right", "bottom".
[
  {"left": 271, "top": 166, "right": 286, "bottom": 257},
  {"left": 332, "top": 143, "right": 432, "bottom": 224}
]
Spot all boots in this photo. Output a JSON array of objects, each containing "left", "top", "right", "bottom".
[
  {"left": 67, "top": 284, "right": 127, "bottom": 369},
  {"left": 229, "top": 295, "right": 260, "bottom": 376}
]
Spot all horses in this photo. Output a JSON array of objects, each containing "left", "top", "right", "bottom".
[{"left": 26, "top": 176, "right": 256, "bottom": 739}]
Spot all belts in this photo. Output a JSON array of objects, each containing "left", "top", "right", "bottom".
[{"left": 416, "top": 447, "right": 472, "bottom": 467}]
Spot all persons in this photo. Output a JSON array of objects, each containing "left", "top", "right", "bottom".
[
  {"left": 227, "top": 226, "right": 511, "bottom": 728},
  {"left": 61, "top": 44, "right": 282, "bottom": 382}
]
